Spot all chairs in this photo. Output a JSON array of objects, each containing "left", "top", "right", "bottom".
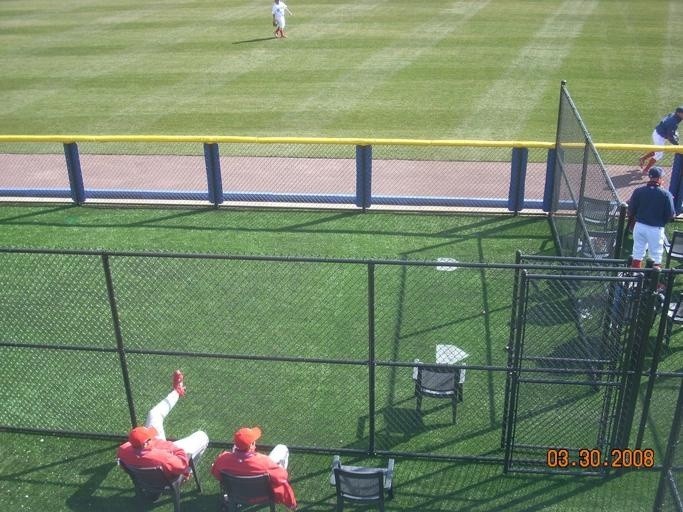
[
  {"left": 116, "top": 454, "right": 202, "bottom": 510},
  {"left": 659, "top": 232, "right": 683, "bottom": 344},
  {"left": 328, "top": 454, "right": 395, "bottom": 511},
  {"left": 578, "top": 197, "right": 619, "bottom": 263},
  {"left": 218, "top": 471, "right": 276, "bottom": 511},
  {"left": 410, "top": 358, "right": 463, "bottom": 425}
]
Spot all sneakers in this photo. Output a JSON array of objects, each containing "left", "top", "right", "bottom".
[{"left": 173, "top": 370, "right": 186, "bottom": 398}]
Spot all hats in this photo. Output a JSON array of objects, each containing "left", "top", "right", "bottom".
[
  {"left": 648, "top": 166, "right": 666, "bottom": 179},
  {"left": 128, "top": 426, "right": 158, "bottom": 447},
  {"left": 236, "top": 426, "right": 261, "bottom": 447}
]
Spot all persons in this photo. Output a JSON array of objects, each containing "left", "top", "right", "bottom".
[
  {"left": 639, "top": 107, "right": 683, "bottom": 174},
  {"left": 117, "top": 370, "right": 209, "bottom": 481},
  {"left": 626, "top": 166, "right": 675, "bottom": 292},
  {"left": 210, "top": 426, "right": 297, "bottom": 509},
  {"left": 272, "top": 0, "right": 293, "bottom": 38}
]
